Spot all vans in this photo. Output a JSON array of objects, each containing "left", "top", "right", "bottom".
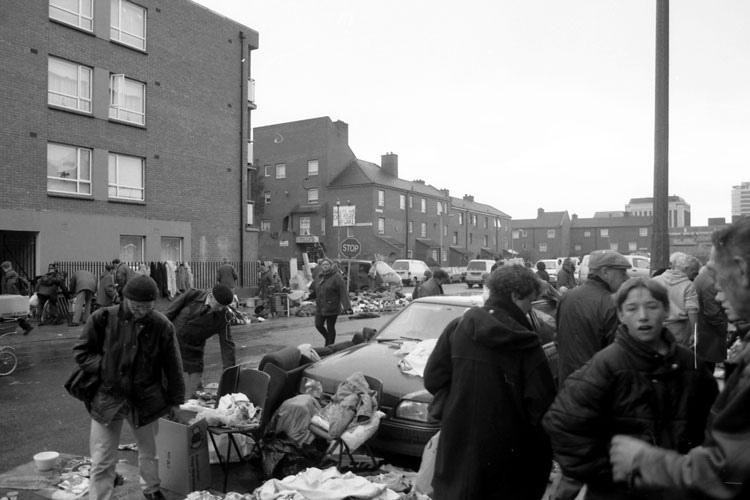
[
  {"left": 342, "top": 260, "right": 402, "bottom": 290},
  {"left": 392, "top": 259, "right": 434, "bottom": 286},
  {"left": 462, "top": 259, "right": 497, "bottom": 289},
  {"left": 580, "top": 253, "right": 650, "bottom": 286}
]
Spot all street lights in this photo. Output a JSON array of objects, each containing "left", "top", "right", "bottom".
[{"left": 335, "top": 197, "right": 342, "bottom": 260}]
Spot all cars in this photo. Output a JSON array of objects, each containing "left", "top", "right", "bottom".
[
  {"left": 298, "top": 292, "right": 558, "bottom": 459},
  {"left": 441, "top": 266, "right": 464, "bottom": 284},
  {"left": 529, "top": 250, "right": 620, "bottom": 285}
]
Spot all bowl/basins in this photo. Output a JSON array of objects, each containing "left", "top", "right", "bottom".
[{"left": 33, "top": 450, "right": 58, "bottom": 471}]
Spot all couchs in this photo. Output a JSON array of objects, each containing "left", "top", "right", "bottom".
[{"left": 259, "top": 333, "right": 366, "bottom": 407}]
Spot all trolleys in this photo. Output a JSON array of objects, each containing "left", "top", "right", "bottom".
[{"left": 0, "top": 317, "right": 21, "bottom": 377}]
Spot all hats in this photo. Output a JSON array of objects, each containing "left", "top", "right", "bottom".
[
  {"left": 123, "top": 275, "right": 159, "bottom": 302},
  {"left": 588, "top": 250, "right": 632, "bottom": 271},
  {"left": 212, "top": 286, "right": 233, "bottom": 305}
]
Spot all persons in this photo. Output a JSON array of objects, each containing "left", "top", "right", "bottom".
[
  {"left": 35, "top": 271, "right": 70, "bottom": 326},
  {"left": 72, "top": 274, "right": 186, "bottom": 500},
  {"left": 258, "top": 266, "right": 272, "bottom": 289},
  {"left": 424, "top": 216, "right": 750, "bottom": 500},
  {"left": 70, "top": 270, "right": 96, "bottom": 326},
  {"left": 96, "top": 259, "right": 132, "bottom": 310},
  {"left": 161, "top": 285, "right": 235, "bottom": 393},
  {"left": 307, "top": 259, "right": 353, "bottom": 347},
  {"left": 217, "top": 257, "right": 238, "bottom": 289},
  {"left": 2, "top": 261, "right": 34, "bottom": 336},
  {"left": 412, "top": 270, "right": 448, "bottom": 300}
]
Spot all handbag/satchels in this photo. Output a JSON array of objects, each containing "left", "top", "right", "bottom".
[
  {"left": 65, "top": 369, "right": 98, "bottom": 401},
  {"left": 427, "top": 386, "right": 449, "bottom": 424}
]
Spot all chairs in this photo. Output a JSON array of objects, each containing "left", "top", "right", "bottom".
[
  {"left": 215, "top": 364, "right": 240, "bottom": 408},
  {"left": 325, "top": 376, "right": 384, "bottom": 474},
  {"left": 207, "top": 368, "right": 271, "bottom": 494}
]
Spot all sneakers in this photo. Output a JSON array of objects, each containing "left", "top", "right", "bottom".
[{"left": 144, "top": 491, "right": 166, "bottom": 500}]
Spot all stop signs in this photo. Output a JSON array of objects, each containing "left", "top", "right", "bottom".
[{"left": 340, "top": 236, "right": 362, "bottom": 259}]
